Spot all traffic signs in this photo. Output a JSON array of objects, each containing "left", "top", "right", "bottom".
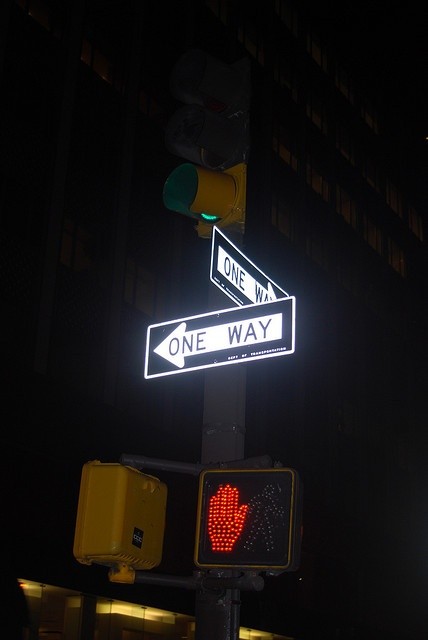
[
  {"left": 144, "top": 296, "right": 296, "bottom": 379},
  {"left": 209, "top": 225, "right": 290, "bottom": 308}
]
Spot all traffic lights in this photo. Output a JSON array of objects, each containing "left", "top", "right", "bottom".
[
  {"left": 162, "top": 159, "right": 250, "bottom": 236},
  {"left": 198, "top": 470, "right": 292, "bottom": 566}
]
